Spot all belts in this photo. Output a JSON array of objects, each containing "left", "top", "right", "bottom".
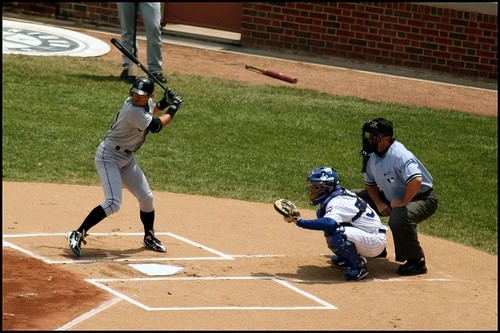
[
  {"left": 115, "top": 146, "right": 132, "bottom": 154},
  {"left": 378, "top": 228, "right": 386, "bottom": 233}
]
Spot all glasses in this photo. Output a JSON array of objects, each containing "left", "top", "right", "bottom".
[{"left": 366, "top": 133, "right": 390, "bottom": 143}]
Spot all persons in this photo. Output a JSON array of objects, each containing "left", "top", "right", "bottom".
[
  {"left": 65, "top": 76, "right": 183, "bottom": 256},
  {"left": 350, "top": 117, "right": 438, "bottom": 276},
  {"left": 117, "top": 2, "right": 166, "bottom": 83},
  {"left": 284, "top": 167, "right": 388, "bottom": 281}
]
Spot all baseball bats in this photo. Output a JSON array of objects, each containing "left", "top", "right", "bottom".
[
  {"left": 110, "top": 37, "right": 169, "bottom": 96},
  {"left": 245, "top": 61, "right": 297, "bottom": 85}
]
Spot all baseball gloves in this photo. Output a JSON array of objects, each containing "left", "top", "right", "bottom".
[{"left": 273, "top": 199, "right": 302, "bottom": 222}]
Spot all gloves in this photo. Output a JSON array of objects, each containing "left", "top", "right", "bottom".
[
  {"left": 156, "top": 86, "right": 175, "bottom": 111},
  {"left": 164, "top": 95, "right": 184, "bottom": 119}
]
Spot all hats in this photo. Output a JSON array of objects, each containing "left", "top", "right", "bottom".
[
  {"left": 362, "top": 118, "right": 393, "bottom": 136},
  {"left": 130, "top": 77, "right": 154, "bottom": 96}
]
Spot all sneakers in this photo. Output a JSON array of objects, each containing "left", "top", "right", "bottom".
[
  {"left": 331, "top": 255, "right": 351, "bottom": 270},
  {"left": 65, "top": 229, "right": 90, "bottom": 258},
  {"left": 397, "top": 257, "right": 427, "bottom": 276},
  {"left": 121, "top": 69, "right": 135, "bottom": 82},
  {"left": 345, "top": 258, "right": 369, "bottom": 281},
  {"left": 152, "top": 72, "right": 167, "bottom": 83},
  {"left": 144, "top": 230, "right": 165, "bottom": 253}
]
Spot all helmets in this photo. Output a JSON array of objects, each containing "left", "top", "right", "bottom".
[{"left": 306, "top": 166, "right": 342, "bottom": 195}]
